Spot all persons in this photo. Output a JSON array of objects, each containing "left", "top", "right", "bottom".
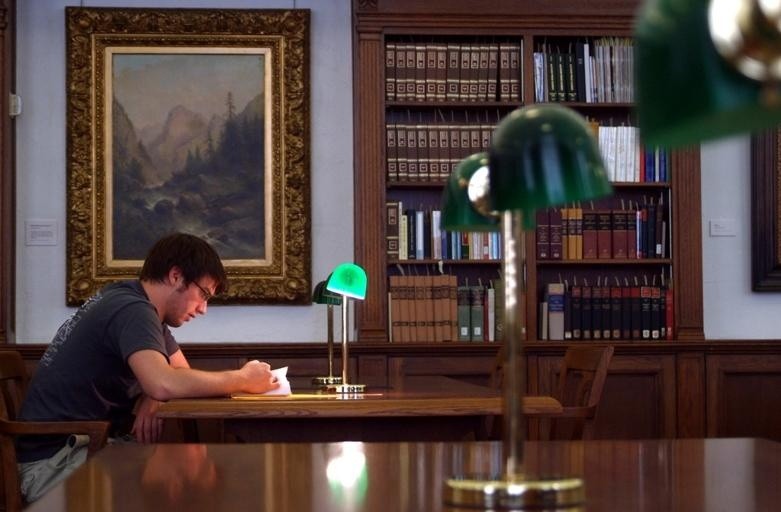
[{"left": 15, "top": 232, "right": 279, "bottom": 502}]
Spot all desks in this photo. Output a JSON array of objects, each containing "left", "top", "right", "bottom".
[
  {"left": 156, "top": 374, "right": 565, "bottom": 441},
  {"left": 19, "top": 437, "right": 780, "bottom": 511}
]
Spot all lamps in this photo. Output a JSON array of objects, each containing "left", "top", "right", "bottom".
[
  {"left": 309, "top": 261, "right": 369, "bottom": 395},
  {"left": 630, "top": 2, "right": 781, "bottom": 152},
  {"left": 438, "top": 101, "right": 617, "bottom": 512}
]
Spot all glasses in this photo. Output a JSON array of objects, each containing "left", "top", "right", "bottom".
[{"left": 190, "top": 277, "right": 212, "bottom": 302}]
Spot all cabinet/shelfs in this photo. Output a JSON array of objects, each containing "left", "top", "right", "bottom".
[{"left": 351, "top": 0, "right": 707, "bottom": 439}]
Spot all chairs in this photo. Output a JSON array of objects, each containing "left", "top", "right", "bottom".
[
  {"left": 0, "top": 346, "right": 109, "bottom": 512},
  {"left": 524, "top": 343, "right": 618, "bottom": 439}
]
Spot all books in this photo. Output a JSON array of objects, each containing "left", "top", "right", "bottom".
[
  {"left": 536, "top": 190, "right": 665, "bottom": 260},
  {"left": 387, "top": 41, "right": 521, "bottom": 102},
  {"left": 229, "top": 367, "right": 291, "bottom": 398},
  {"left": 538, "top": 271, "right": 673, "bottom": 340},
  {"left": 386, "top": 107, "right": 508, "bottom": 182},
  {"left": 586, "top": 113, "right": 669, "bottom": 183},
  {"left": 387, "top": 199, "right": 501, "bottom": 262},
  {"left": 390, "top": 275, "right": 505, "bottom": 344},
  {"left": 533, "top": 39, "right": 633, "bottom": 102}
]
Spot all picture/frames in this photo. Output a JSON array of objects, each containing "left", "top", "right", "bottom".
[{"left": 64, "top": 7, "right": 314, "bottom": 306}]
